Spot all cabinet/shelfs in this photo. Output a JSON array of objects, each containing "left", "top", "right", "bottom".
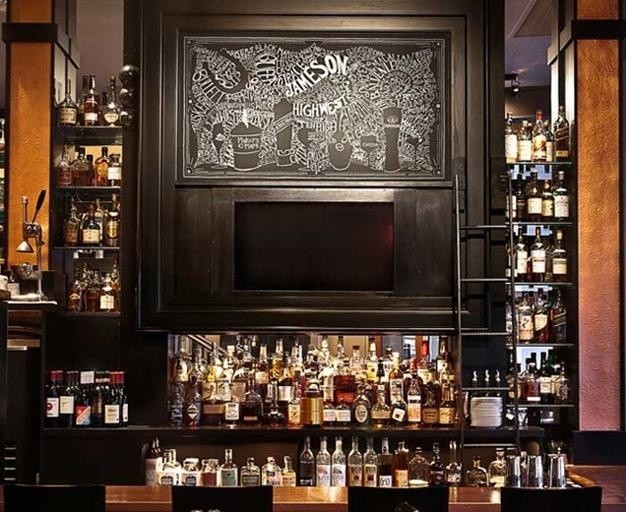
[
  {"left": 506, "top": 127, "right": 576, "bottom": 481},
  {"left": 52, "top": 334, "right": 456, "bottom": 484},
  {"left": 453, "top": 178, "right": 504, "bottom": 479},
  {"left": 45, "top": 121, "right": 124, "bottom": 319}
]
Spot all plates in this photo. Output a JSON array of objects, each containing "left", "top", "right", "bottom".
[{"left": 468, "top": 397, "right": 504, "bottom": 427}]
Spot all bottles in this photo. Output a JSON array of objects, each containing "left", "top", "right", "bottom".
[
  {"left": 142, "top": 434, "right": 508, "bottom": 487},
  {"left": 45, "top": 369, "right": 130, "bottom": 428},
  {"left": 505, "top": 109, "right": 570, "bottom": 406},
  {"left": 57, "top": 73, "right": 123, "bottom": 316},
  {"left": 171, "top": 333, "right": 456, "bottom": 428}
]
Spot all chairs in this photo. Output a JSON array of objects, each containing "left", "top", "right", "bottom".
[
  {"left": 500, "top": 485, "right": 605, "bottom": 512},
  {"left": 172, "top": 484, "right": 274, "bottom": 512},
  {"left": 346, "top": 484, "right": 450, "bottom": 512},
  {"left": 2, "top": 481, "right": 107, "bottom": 511}
]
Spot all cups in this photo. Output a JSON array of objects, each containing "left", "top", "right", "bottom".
[{"left": 507, "top": 454, "right": 569, "bottom": 488}]
